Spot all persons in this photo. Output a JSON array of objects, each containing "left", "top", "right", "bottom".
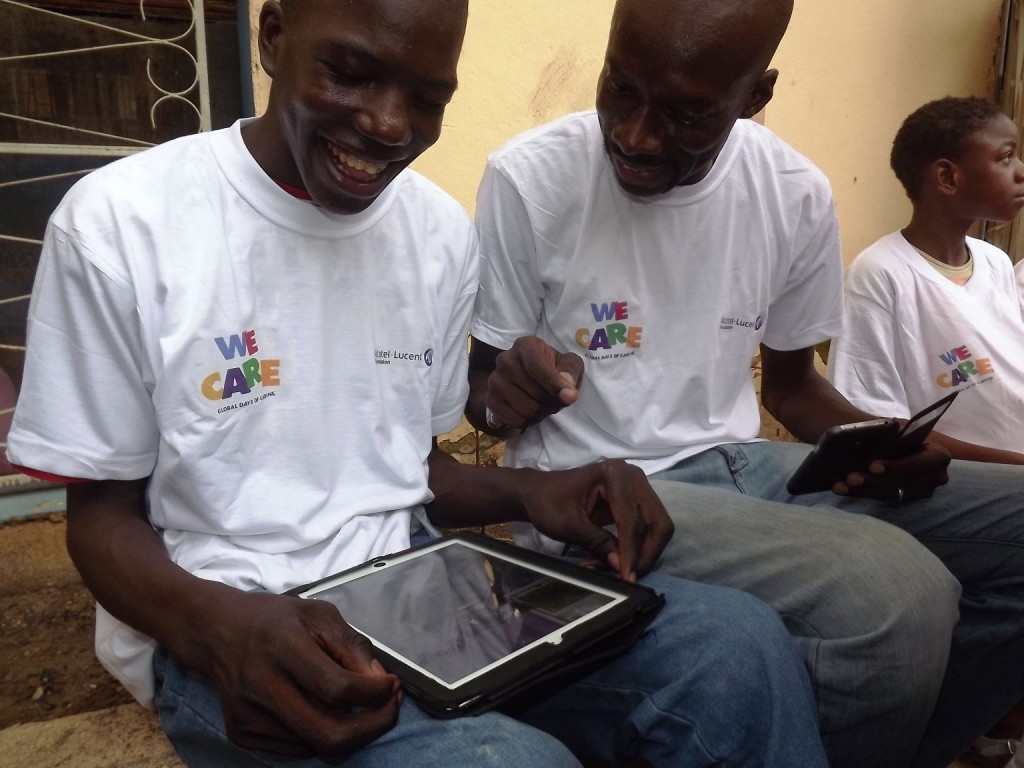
[
  {"left": 3, "top": 1, "right": 830, "bottom": 768},
  {"left": 463, "top": 0, "right": 1024, "bottom": 768},
  {"left": 828, "top": 96, "right": 1024, "bottom": 768}
]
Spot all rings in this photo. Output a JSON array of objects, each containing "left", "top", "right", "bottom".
[{"left": 895, "top": 488, "right": 903, "bottom": 503}]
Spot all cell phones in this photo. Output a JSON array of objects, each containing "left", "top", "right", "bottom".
[{"left": 785, "top": 416, "right": 901, "bottom": 497}]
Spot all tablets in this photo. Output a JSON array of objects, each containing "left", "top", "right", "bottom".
[{"left": 276, "top": 529, "right": 665, "bottom": 723}]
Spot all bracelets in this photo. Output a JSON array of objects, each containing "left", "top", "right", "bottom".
[{"left": 486, "top": 407, "right": 528, "bottom": 434}]
